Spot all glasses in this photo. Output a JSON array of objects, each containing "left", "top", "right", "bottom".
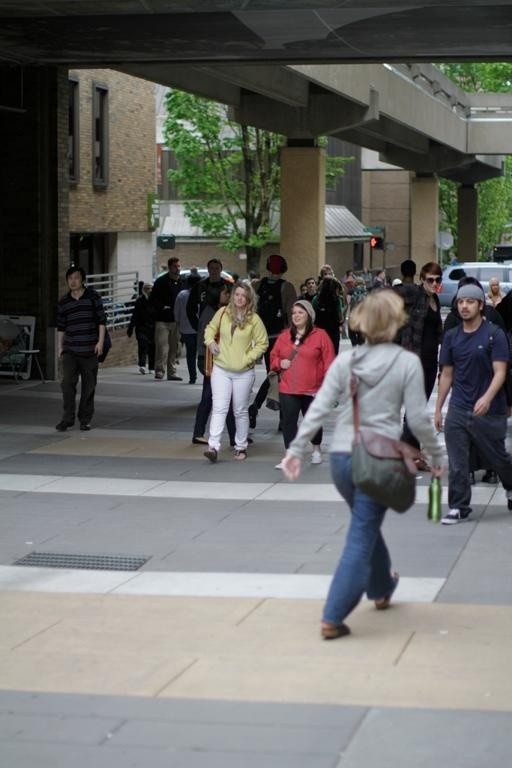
[{"left": 421, "top": 276, "right": 440, "bottom": 282}]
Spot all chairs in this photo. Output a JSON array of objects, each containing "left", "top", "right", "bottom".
[{"left": 0, "top": 318, "right": 46, "bottom": 386}]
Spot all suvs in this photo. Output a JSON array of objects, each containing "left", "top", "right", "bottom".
[
  {"left": 435, "top": 260, "right": 512, "bottom": 310},
  {"left": 154, "top": 267, "right": 234, "bottom": 285}
]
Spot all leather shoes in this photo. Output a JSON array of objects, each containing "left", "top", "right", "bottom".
[
  {"left": 191, "top": 404, "right": 257, "bottom": 462},
  {"left": 467, "top": 468, "right": 496, "bottom": 483}
]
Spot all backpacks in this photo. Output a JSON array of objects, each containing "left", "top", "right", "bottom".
[{"left": 257, "top": 285, "right": 288, "bottom": 334}]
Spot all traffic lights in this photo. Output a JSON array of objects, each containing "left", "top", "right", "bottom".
[{"left": 369, "top": 236, "right": 385, "bottom": 250}]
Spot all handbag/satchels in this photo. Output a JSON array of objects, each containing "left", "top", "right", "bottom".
[
  {"left": 91, "top": 325, "right": 114, "bottom": 361},
  {"left": 351, "top": 429, "right": 425, "bottom": 514},
  {"left": 205, "top": 346, "right": 214, "bottom": 377},
  {"left": 265, "top": 370, "right": 280, "bottom": 410}
]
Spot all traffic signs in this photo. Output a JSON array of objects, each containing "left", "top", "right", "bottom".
[{"left": 363, "top": 225, "right": 384, "bottom": 233}]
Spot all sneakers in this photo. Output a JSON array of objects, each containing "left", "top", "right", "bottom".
[
  {"left": 138, "top": 366, "right": 200, "bottom": 384},
  {"left": 440, "top": 505, "right": 470, "bottom": 523},
  {"left": 56, "top": 414, "right": 72, "bottom": 431},
  {"left": 373, "top": 572, "right": 399, "bottom": 609},
  {"left": 310, "top": 450, "right": 321, "bottom": 464},
  {"left": 80, "top": 417, "right": 91, "bottom": 428},
  {"left": 321, "top": 620, "right": 351, "bottom": 639}
]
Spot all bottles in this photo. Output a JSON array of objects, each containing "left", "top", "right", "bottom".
[{"left": 426, "top": 473, "right": 442, "bottom": 522}]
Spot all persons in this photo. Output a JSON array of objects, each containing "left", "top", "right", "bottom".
[
  {"left": 391, "top": 259, "right": 512, "bottom": 524},
  {"left": 55, "top": 267, "right": 107, "bottom": 432},
  {"left": 298, "top": 264, "right": 392, "bottom": 354},
  {"left": 279, "top": 288, "right": 449, "bottom": 639},
  {"left": 126, "top": 257, "right": 201, "bottom": 385},
  {"left": 185, "top": 256, "right": 296, "bottom": 461}
]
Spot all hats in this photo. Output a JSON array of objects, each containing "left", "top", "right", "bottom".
[
  {"left": 454, "top": 284, "right": 482, "bottom": 300},
  {"left": 265, "top": 254, "right": 287, "bottom": 271},
  {"left": 294, "top": 298, "right": 314, "bottom": 320}
]
